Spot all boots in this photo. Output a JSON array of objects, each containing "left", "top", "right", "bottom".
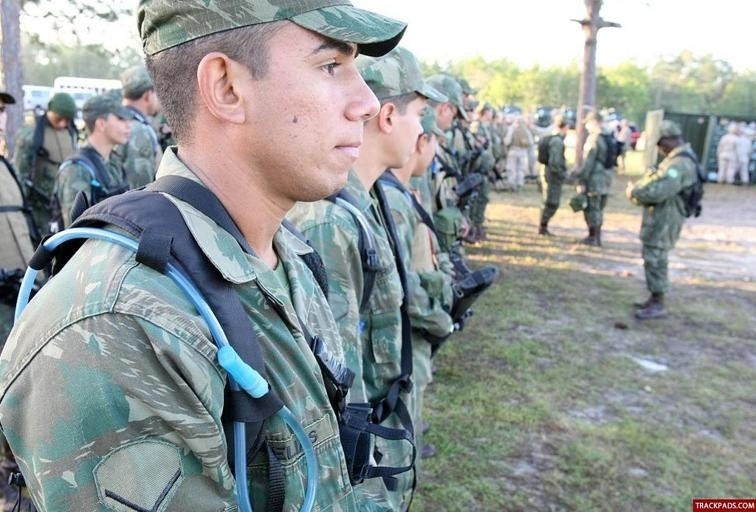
[
  {"left": 636, "top": 291, "right": 669, "bottom": 318},
  {"left": 580, "top": 225, "right": 602, "bottom": 246},
  {"left": 538, "top": 223, "right": 556, "bottom": 238},
  {"left": 634, "top": 293, "right": 653, "bottom": 308}
]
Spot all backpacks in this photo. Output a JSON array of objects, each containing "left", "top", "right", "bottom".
[
  {"left": 668, "top": 151, "right": 707, "bottom": 220},
  {"left": 537, "top": 133, "right": 562, "bottom": 166},
  {"left": 597, "top": 132, "right": 621, "bottom": 170}
]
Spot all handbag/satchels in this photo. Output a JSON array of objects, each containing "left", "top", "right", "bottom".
[
  {"left": 569, "top": 194, "right": 591, "bottom": 214},
  {"left": 431, "top": 204, "right": 472, "bottom": 253}
]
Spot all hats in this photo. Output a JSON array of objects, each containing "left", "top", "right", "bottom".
[
  {"left": 118, "top": 64, "right": 152, "bottom": 95},
  {"left": 453, "top": 76, "right": 479, "bottom": 96},
  {"left": 465, "top": 99, "right": 480, "bottom": 110},
  {"left": 647, "top": 119, "right": 684, "bottom": 148},
  {"left": 419, "top": 104, "right": 447, "bottom": 140},
  {"left": 0, "top": 92, "right": 17, "bottom": 107},
  {"left": 46, "top": 90, "right": 80, "bottom": 121},
  {"left": 351, "top": 45, "right": 450, "bottom": 107},
  {"left": 135, "top": 0, "right": 410, "bottom": 67},
  {"left": 81, "top": 89, "right": 135, "bottom": 121},
  {"left": 424, "top": 74, "right": 471, "bottom": 125}
]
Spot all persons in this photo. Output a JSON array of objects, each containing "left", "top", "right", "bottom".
[
  {"left": 717, "top": 125, "right": 740, "bottom": 184},
  {"left": 570, "top": 111, "right": 614, "bottom": 248},
  {"left": 737, "top": 129, "right": 753, "bottom": 184},
  {"left": 625, "top": 119, "right": 699, "bottom": 319},
  {"left": 613, "top": 120, "right": 631, "bottom": 166}
]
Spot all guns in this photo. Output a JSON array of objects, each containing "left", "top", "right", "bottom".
[
  {"left": 455, "top": 173, "right": 481, "bottom": 213},
  {"left": 453, "top": 267, "right": 495, "bottom": 320}
]
